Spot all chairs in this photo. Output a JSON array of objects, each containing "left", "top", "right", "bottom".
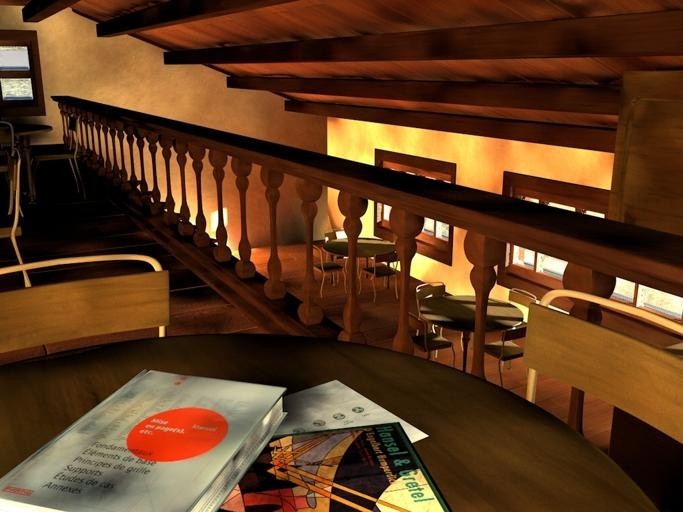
[
  {"left": 312, "top": 231, "right": 399, "bottom": 303},
  {"left": 28, "top": 117, "right": 87, "bottom": 201},
  {"left": 2, "top": 253, "right": 170, "bottom": 367},
  {"left": 409, "top": 281, "right": 537, "bottom": 387},
  {"left": 1, "top": 117, "right": 54, "bottom": 291}
]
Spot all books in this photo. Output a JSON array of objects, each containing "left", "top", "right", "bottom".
[
  {"left": 0, "top": 365, "right": 289, "bottom": 512},
  {"left": 239, "top": 421, "right": 456, "bottom": 512}
]
